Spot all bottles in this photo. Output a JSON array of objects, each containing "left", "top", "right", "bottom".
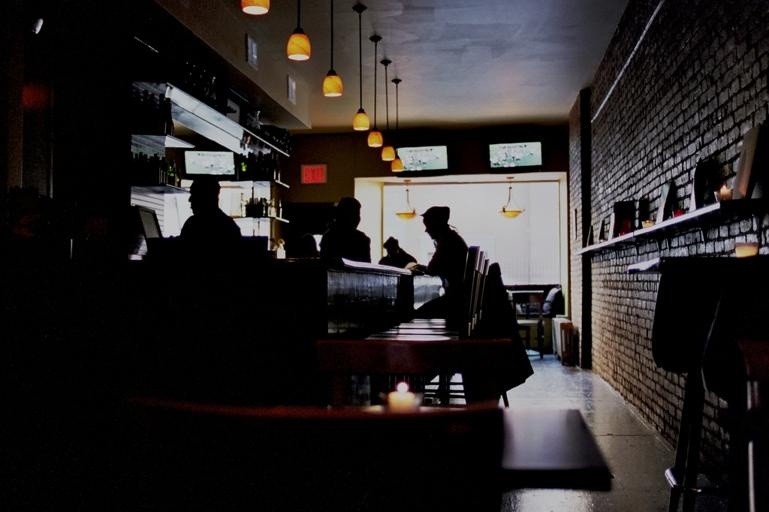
[{"left": 422, "top": 206, "right": 450, "bottom": 225}]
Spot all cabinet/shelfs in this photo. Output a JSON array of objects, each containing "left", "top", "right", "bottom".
[
  {"left": 245, "top": 198, "right": 282, "bottom": 218},
  {"left": 129, "top": 151, "right": 182, "bottom": 188},
  {"left": 237, "top": 151, "right": 281, "bottom": 182},
  {"left": 136, "top": 88, "right": 175, "bottom": 137}
]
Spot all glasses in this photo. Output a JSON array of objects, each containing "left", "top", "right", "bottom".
[
  {"left": 241, "top": 0, "right": 270, "bottom": 15},
  {"left": 367, "top": 34, "right": 384, "bottom": 148},
  {"left": 396, "top": 180, "right": 417, "bottom": 219},
  {"left": 353, "top": 0, "right": 370, "bottom": 131},
  {"left": 380, "top": 56, "right": 396, "bottom": 162},
  {"left": 501, "top": 177, "right": 525, "bottom": 218},
  {"left": 322, "top": 0, "right": 344, "bottom": 97},
  {"left": 286, "top": 0, "right": 312, "bottom": 61},
  {"left": 391, "top": 77, "right": 403, "bottom": 172}
]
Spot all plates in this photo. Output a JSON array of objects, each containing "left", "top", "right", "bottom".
[
  {"left": 551, "top": 318, "right": 573, "bottom": 365},
  {"left": 242, "top": 128, "right": 290, "bottom": 225}
]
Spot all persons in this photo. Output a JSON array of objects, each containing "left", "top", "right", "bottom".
[
  {"left": 179, "top": 180, "right": 242, "bottom": 237},
  {"left": 410, "top": 206, "right": 468, "bottom": 319},
  {"left": 379, "top": 237, "right": 417, "bottom": 269},
  {"left": 320, "top": 197, "right": 371, "bottom": 263}
]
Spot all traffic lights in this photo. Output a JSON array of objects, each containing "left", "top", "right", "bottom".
[{"left": 543, "top": 286, "right": 562, "bottom": 312}]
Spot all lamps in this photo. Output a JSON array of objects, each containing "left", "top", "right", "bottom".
[
  {"left": 512, "top": 293, "right": 545, "bottom": 358},
  {"left": 361, "top": 246, "right": 511, "bottom": 409},
  {"left": 650, "top": 255, "right": 769, "bottom": 512},
  {"left": 129, "top": 398, "right": 504, "bottom": 512}
]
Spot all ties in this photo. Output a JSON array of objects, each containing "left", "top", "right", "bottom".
[
  {"left": 396, "top": 145, "right": 449, "bottom": 174},
  {"left": 488, "top": 140, "right": 543, "bottom": 169},
  {"left": 183, "top": 149, "right": 239, "bottom": 180},
  {"left": 134, "top": 204, "right": 162, "bottom": 239}
]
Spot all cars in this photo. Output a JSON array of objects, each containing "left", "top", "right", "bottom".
[
  {"left": 734, "top": 244, "right": 759, "bottom": 257},
  {"left": 714, "top": 189, "right": 733, "bottom": 203},
  {"left": 642, "top": 220, "right": 654, "bottom": 229}
]
